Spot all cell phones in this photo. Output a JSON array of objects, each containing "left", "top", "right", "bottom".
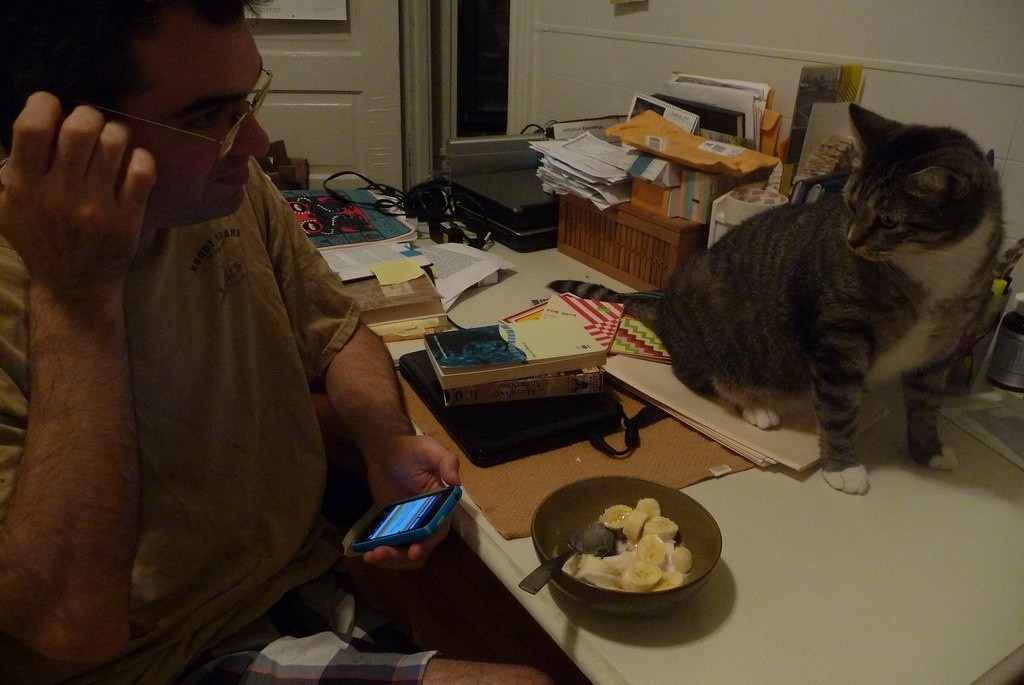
[{"left": 351, "top": 486, "right": 461, "bottom": 552}]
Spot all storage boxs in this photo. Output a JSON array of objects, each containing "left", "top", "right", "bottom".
[
  {"left": 557, "top": 193, "right": 708, "bottom": 292},
  {"left": 267, "top": 139, "right": 310, "bottom": 190},
  {"left": 630, "top": 179, "right": 670, "bottom": 215}
]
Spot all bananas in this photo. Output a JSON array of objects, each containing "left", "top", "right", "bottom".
[{"left": 563, "top": 496, "right": 693, "bottom": 596}]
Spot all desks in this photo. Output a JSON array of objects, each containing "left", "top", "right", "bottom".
[{"left": 340, "top": 215, "right": 1024, "bottom": 684}]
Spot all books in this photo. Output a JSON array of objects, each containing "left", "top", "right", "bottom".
[
  {"left": 653, "top": 94, "right": 746, "bottom": 137},
  {"left": 421, "top": 318, "right": 606, "bottom": 407},
  {"left": 343, "top": 269, "right": 449, "bottom": 342}
]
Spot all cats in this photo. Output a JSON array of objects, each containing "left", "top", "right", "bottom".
[{"left": 544, "top": 102, "right": 1008, "bottom": 496}]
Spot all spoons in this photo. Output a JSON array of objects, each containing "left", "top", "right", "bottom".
[{"left": 518, "top": 521, "right": 615, "bottom": 595}]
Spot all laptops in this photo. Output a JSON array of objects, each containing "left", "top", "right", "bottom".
[
  {"left": 451, "top": 168, "right": 562, "bottom": 251},
  {"left": 398, "top": 336, "right": 622, "bottom": 465}
]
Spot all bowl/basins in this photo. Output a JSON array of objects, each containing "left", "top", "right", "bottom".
[{"left": 530, "top": 476, "right": 723, "bottom": 617}]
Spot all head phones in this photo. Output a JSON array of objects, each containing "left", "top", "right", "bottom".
[{"left": 404, "top": 179, "right": 448, "bottom": 217}]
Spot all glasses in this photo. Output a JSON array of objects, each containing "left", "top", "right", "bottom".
[{"left": 68, "top": 72, "right": 273, "bottom": 159}]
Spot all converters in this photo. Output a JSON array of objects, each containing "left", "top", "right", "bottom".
[{"left": 428, "top": 218, "right": 463, "bottom": 246}]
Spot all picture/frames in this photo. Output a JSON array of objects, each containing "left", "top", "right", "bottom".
[{"left": 244, "top": 0, "right": 350, "bottom": 24}]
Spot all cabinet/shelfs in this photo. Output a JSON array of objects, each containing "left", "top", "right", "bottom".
[{"left": 707, "top": 189, "right": 730, "bottom": 250}]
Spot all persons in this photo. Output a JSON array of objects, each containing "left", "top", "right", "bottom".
[{"left": 0, "top": 0, "right": 576, "bottom": 685}]
